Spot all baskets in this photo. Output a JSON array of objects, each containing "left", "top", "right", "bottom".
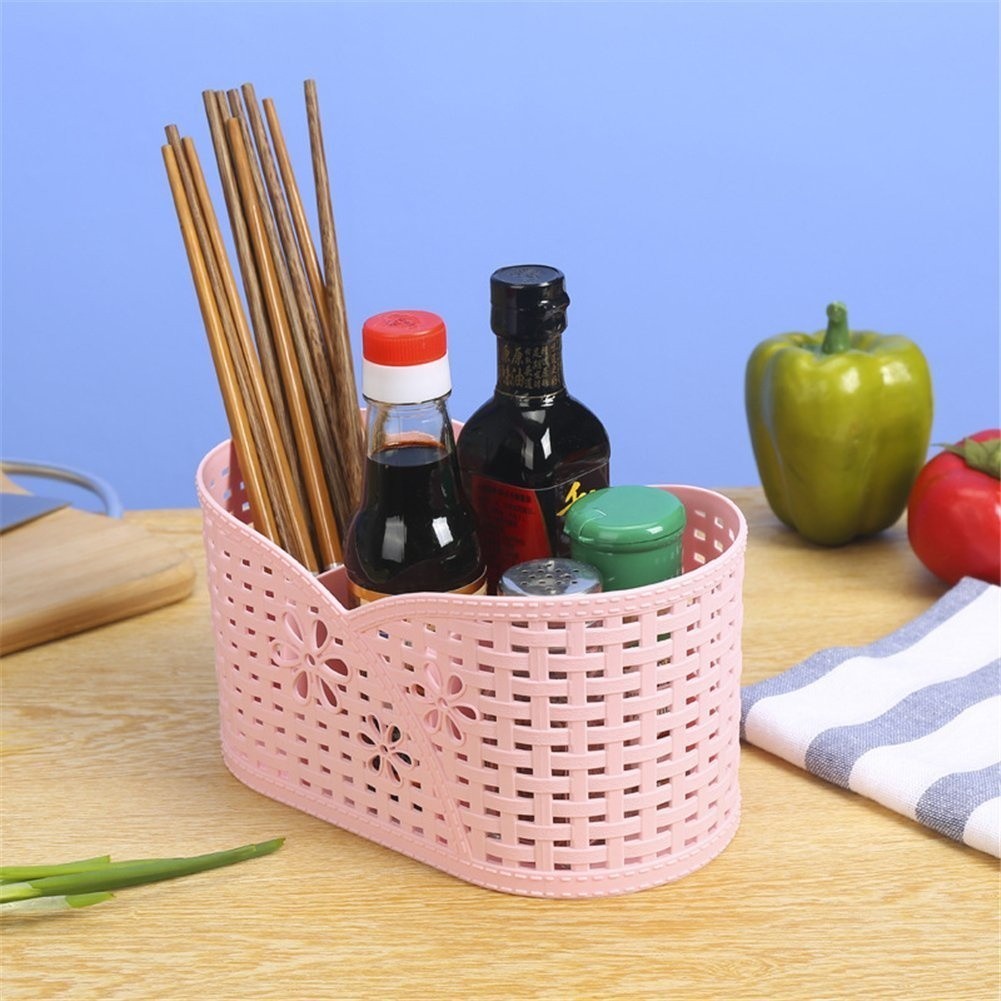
[{"left": 192, "top": 415, "right": 750, "bottom": 900}]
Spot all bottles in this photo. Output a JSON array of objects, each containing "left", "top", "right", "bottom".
[
  {"left": 564, "top": 488, "right": 685, "bottom": 738},
  {"left": 346, "top": 311, "right": 488, "bottom": 811},
  {"left": 499, "top": 560, "right": 604, "bottom": 869},
  {"left": 455, "top": 264, "right": 611, "bottom": 596}
]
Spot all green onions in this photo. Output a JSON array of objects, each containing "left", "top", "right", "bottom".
[{"left": 1, "top": 834, "right": 285, "bottom": 902}]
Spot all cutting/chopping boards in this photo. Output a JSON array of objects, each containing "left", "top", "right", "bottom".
[{"left": 2, "top": 472, "right": 192, "bottom": 662}]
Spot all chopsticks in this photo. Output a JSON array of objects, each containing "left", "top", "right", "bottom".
[{"left": 154, "top": 75, "right": 366, "bottom": 574}]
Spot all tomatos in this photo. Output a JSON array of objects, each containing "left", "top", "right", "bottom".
[{"left": 907, "top": 429, "right": 1001, "bottom": 589}]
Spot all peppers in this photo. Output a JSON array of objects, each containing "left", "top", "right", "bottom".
[{"left": 743, "top": 301, "right": 933, "bottom": 548}]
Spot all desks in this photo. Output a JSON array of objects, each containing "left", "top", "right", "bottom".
[{"left": 3, "top": 493, "right": 1001, "bottom": 999}]
